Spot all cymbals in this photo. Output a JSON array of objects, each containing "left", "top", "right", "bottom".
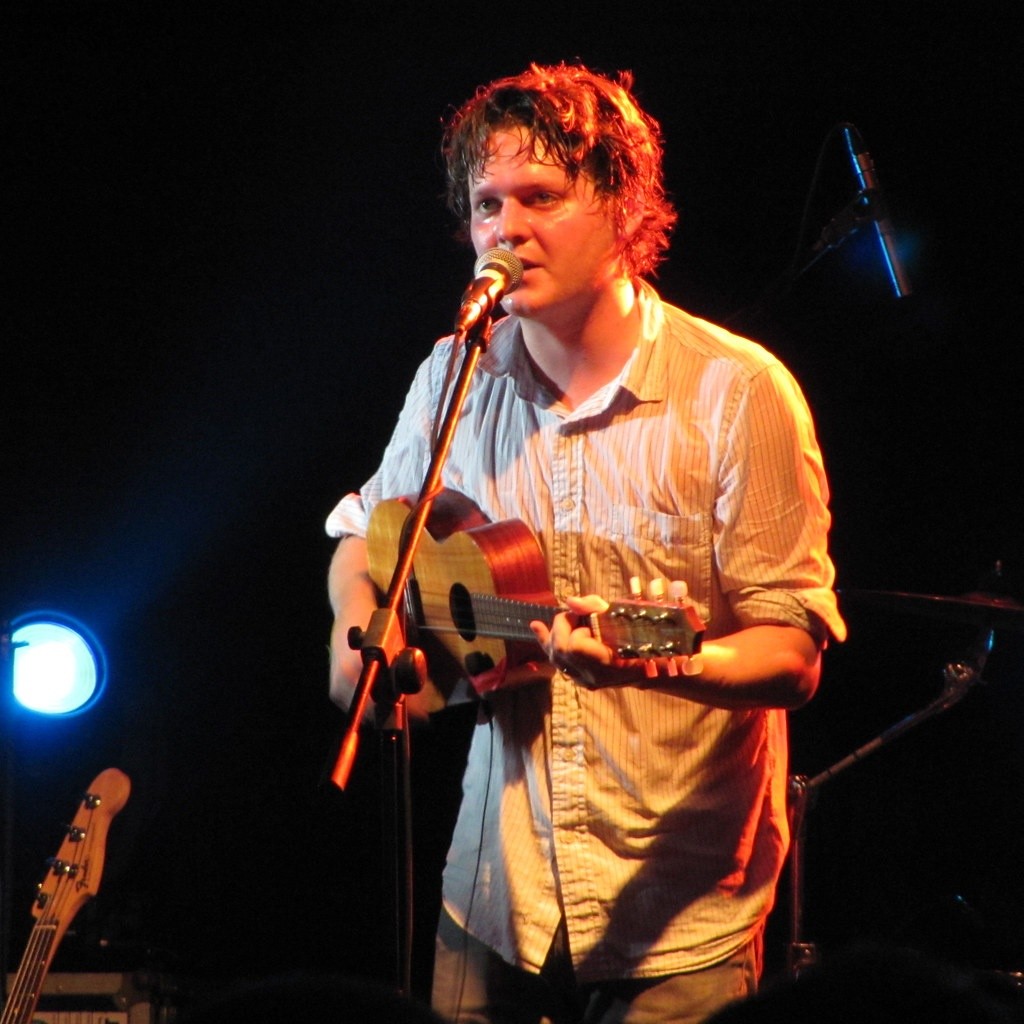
[{"left": 833, "top": 583, "right": 1023, "bottom": 635}]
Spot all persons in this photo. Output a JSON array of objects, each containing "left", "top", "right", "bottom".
[{"left": 321, "top": 66, "right": 849, "bottom": 1024}]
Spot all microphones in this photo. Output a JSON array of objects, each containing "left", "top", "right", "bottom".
[
  {"left": 843, "top": 123, "right": 911, "bottom": 297},
  {"left": 453, "top": 247, "right": 523, "bottom": 335}
]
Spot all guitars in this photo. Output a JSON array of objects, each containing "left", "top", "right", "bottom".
[
  {"left": 362, "top": 486, "right": 707, "bottom": 683},
  {"left": 0, "top": 766, "right": 133, "bottom": 1024}
]
